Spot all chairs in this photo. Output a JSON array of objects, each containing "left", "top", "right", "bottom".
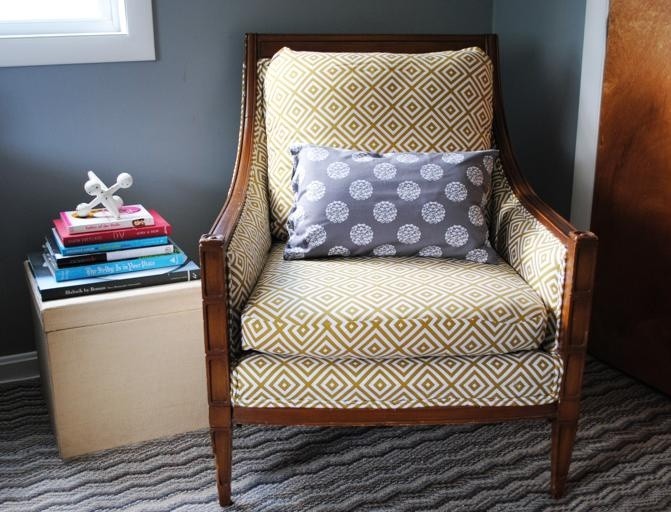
[{"left": 198, "top": 28, "right": 601, "bottom": 507}]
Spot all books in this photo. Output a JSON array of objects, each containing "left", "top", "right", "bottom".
[{"left": 25, "top": 203, "right": 203, "bottom": 303}]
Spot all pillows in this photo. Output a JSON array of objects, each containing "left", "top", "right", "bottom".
[
  {"left": 284, "top": 142, "right": 502, "bottom": 264},
  {"left": 262, "top": 45, "right": 493, "bottom": 243}
]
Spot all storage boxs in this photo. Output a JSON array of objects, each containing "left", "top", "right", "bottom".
[{"left": 18, "top": 251, "right": 211, "bottom": 460}]
